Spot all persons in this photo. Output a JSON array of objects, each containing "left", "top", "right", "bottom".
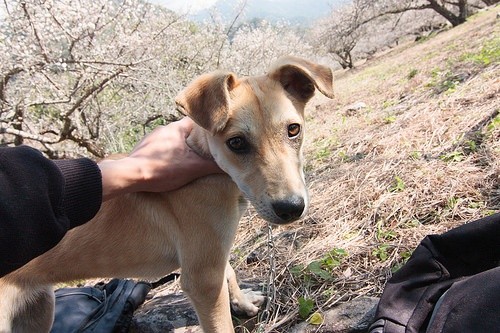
[{"left": 1, "top": 116, "right": 233, "bottom": 277}]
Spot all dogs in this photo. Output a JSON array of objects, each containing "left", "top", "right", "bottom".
[{"left": 0, "top": 55, "right": 335, "bottom": 333}]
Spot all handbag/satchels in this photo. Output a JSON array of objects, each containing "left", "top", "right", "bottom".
[
  {"left": 368, "top": 212, "right": 500, "bottom": 333},
  {"left": 51, "top": 273, "right": 179, "bottom": 333}
]
